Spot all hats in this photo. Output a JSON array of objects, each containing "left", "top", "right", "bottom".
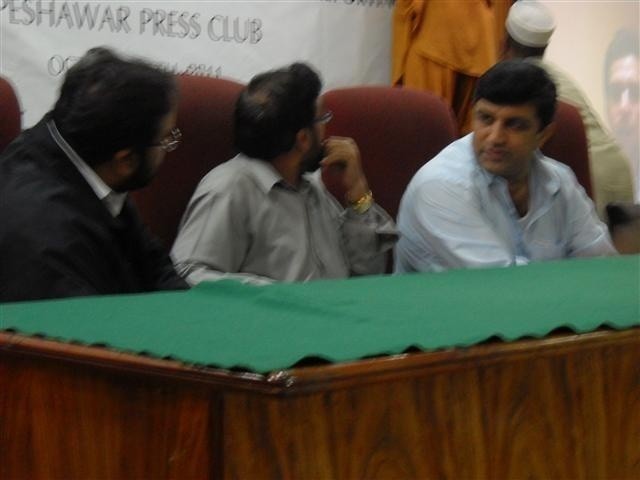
[{"left": 500, "top": 0, "right": 561, "bottom": 51}]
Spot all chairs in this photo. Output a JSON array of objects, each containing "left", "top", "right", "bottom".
[
  {"left": 1, "top": 76, "right": 21, "bottom": 155},
  {"left": 319, "top": 85, "right": 458, "bottom": 220},
  {"left": 539, "top": 104, "right": 592, "bottom": 211},
  {"left": 125, "top": 73, "right": 247, "bottom": 254}
]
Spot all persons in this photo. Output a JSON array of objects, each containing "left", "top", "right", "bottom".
[
  {"left": 391, "top": 0, "right": 513, "bottom": 139},
  {"left": 504, "top": 0, "right": 635, "bottom": 240},
  {"left": 0, "top": 47, "right": 191, "bottom": 305},
  {"left": 168, "top": 62, "right": 401, "bottom": 289},
  {"left": 393, "top": 61, "right": 621, "bottom": 275}
]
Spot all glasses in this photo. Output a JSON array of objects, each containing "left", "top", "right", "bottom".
[
  {"left": 290, "top": 106, "right": 338, "bottom": 135},
  {"left": 118, "top": 124, "right": 187, "bottom": 156}
]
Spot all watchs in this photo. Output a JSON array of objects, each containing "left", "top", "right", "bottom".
[{"left": 345, "top": 190, "right": 373, "bottom": 217}]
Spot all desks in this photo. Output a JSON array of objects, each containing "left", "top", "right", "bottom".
[{"left": 0, "top": 253, "right": 639, "bottom": 479}]
